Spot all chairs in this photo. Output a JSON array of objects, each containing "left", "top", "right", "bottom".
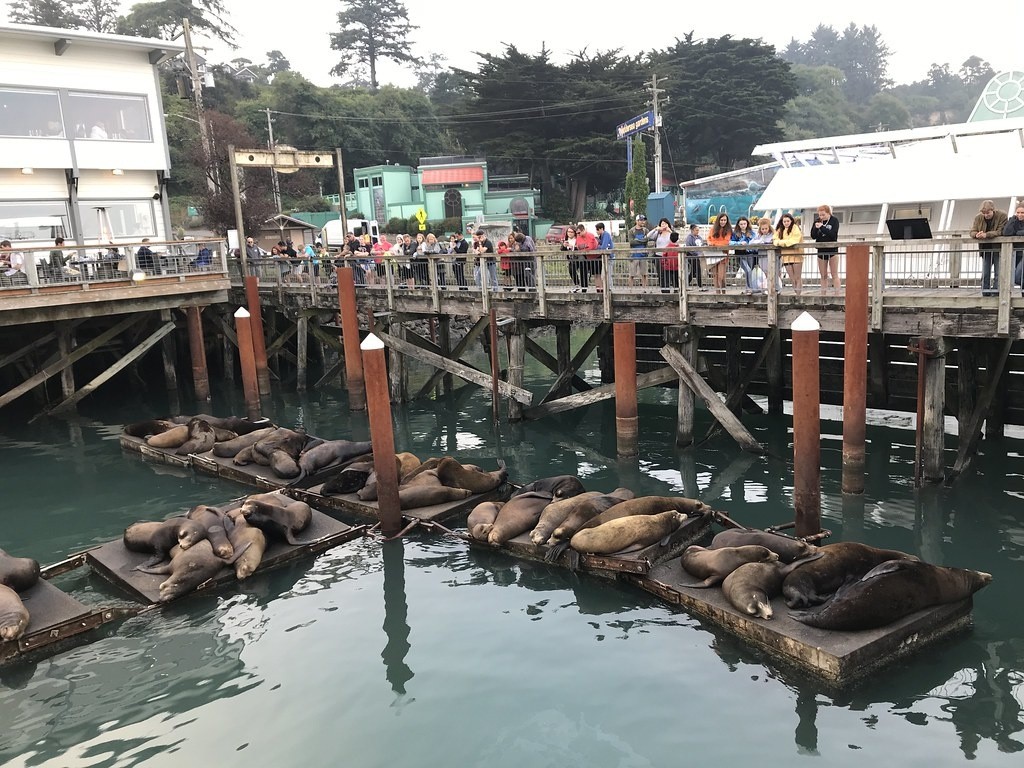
[{"left": 0, "top": 250, "right": 214, "bottom": 287}]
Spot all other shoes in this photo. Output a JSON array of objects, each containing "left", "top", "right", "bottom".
[{"left": 747, "top": 290, "right": 753, "bottom": 295}]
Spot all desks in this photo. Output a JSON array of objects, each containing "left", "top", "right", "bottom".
[
  {"left": 161, "top": 253, "right": 198, "bottom": 273},
  {"left": 70, "top": 259, "right": 119, "bottom": 280}
]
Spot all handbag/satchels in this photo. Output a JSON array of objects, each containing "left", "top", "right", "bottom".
[{"left": 703, "top": 243, "right": 725, "bottom": 265}]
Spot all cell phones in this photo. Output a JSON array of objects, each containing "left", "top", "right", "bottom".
[
  {"left": 816, "top": 217, "right": 820, "bottom": 223},
  {"left": 565, "top": 242, "right": 568, "bottom": 243}
]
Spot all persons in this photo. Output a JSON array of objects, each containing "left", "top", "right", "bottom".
[
  {"left": 749, "top": 218, "right": 782, "bottom": 296},
  {"left": 772, "top": 213, "right": 805, "bottom": 300},
  {"left": 334, "top": 232, "right": 393, "bottom": 287},
  {"left": 560, "top": 220, "right": 616, "bottom": 293},
  {"left": 107, "top": 248, "right": 121, "bottom": 267},
  {"left": 271, "top": 241, "right": 333, "bottom": 287},
  {"left": 661, "top": 232, "right": 681, "bottom": 293},
  {"left": 705, "top": 213, "right": 732, "bottom": 295},
  {"left": 393, "top": 230, "right": 469, "bottom": 292},
  {"left": 0, "top": 240, "right": 28, "bottom": 285},
  {"left": 969, "top": 200, "right": 1008, "bottom": 297},
  {"left": 473, "top": 229, "right": 542, "bottom": 291},
  {"left": 627, "top": 215, "right": 651, "bottom": 295},
  {"left": 46, "top": 116, "right": 141, "bottom": 139},
  {"left": 728, "top": 216, "right": 759, "bottom": 296},
  {"left": 684, "top": 223, "right": 709, "bottom": 292},
  {"left": 811, "top": 204, "right": 842, "bottom": 296},
  {"left": 49, "top": 237, "right": 78, "bottom": 282},
  {"left": 644, "top": 217, "right": 679, "bottom": 295},
  {"left": 228, "top": 232, "right": 262, "bottom": 286},
  {"left": 1003, "top": 202, "right": 1024, "bottom": 297},
  {"left": 189, "top": 243, "right": 210, "bottom": 267},
  {"left": 137, "top": 238, "right": 156, "bottom": 270}
]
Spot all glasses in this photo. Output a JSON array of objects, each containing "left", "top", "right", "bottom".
[
  {"left": 248, "top": 242, "right": 252, "bottom": 243},
  {"left": 576, "top": 232, "right": 580, "bottom": 234},
  {"left": 596, "top": 228, "right": 599, "bottom": 230}
]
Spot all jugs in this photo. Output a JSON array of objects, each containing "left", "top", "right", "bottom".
[
  {"left": 97, "top": 252, "right": 103, "bottom": 260},
  {"left": 177, "top": 246, "right": 183, "bottom": 255}
]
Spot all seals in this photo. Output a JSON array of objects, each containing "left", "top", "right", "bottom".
[
  {"left": 212, "top": 427, "right": 374, "bottom": 486},
  {"left": 0, "top": 548, "right": 39, "bottom": 642},
  {"left": 467, "top": 475, "right": 712, "bottom": 563},
  {"left": 320, "top": 452, "right": 508, "bottom": 511},
  {"left": 124, "top": 493, "right": 319, "bottom": 601},
  {"left": 681, "top": 527, "right": 992, "bottom": 631},
  {"left": 124, "top": 414, "right": 273, "bottom": 455}
]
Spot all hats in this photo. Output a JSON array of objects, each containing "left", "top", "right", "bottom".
[
  {"left": 690, "top": 224, "right": 697, "bottom": 230},
  {"left": 475, "top": 230, "right": 484, "bottom": 235},
  {"left": 636, "top": 215, "right": 647, "bottom": 221}
]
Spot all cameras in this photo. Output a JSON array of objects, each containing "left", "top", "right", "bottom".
[{"left": 663, "top": 227, "right": 666, "bottom": 231}]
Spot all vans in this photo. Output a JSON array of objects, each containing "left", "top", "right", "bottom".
[{"left": 315, "top": 217, "right": 381, "bottom": 249}]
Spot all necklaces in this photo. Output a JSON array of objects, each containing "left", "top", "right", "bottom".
[{"left": 821, "top": 220, "right": 831, "bottom": 227}]
